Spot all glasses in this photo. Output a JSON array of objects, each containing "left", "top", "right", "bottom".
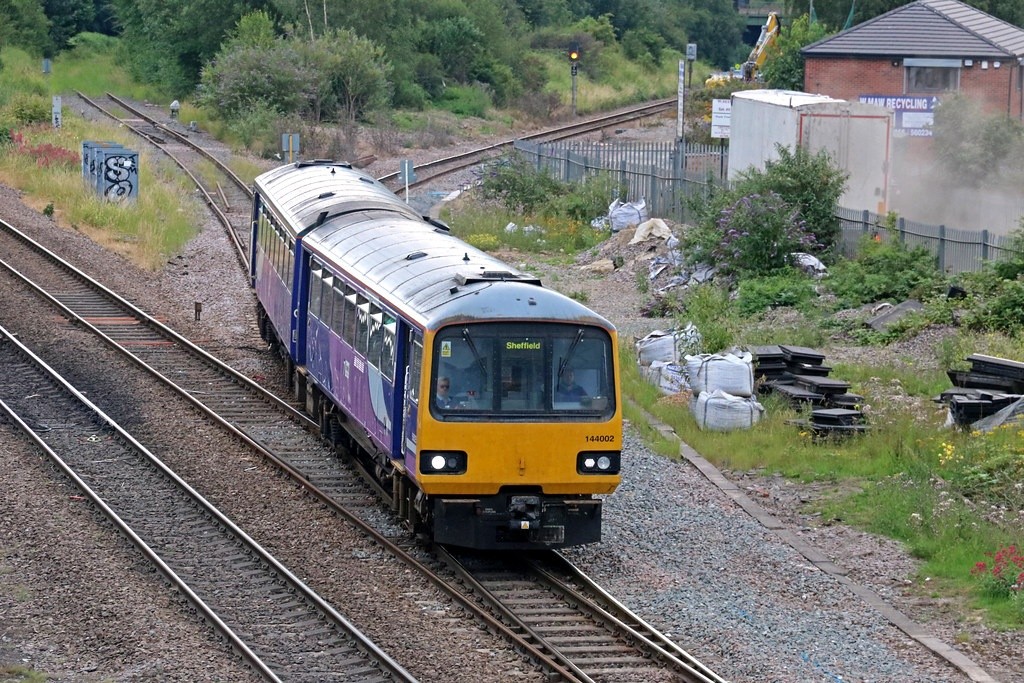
[{"left": 439, "top": 385, "right": 449, "bottom": 389}]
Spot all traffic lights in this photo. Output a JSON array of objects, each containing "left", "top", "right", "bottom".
[{"left": 569, "top": 41, "right": 579, "bottom": 61}]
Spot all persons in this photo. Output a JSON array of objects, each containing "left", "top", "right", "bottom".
[
  {"left": 554, "top": 367, "right": 591, "bottom": 402},
  {"left": 436, "top": 376, "right": 461, "bottom": 410}
]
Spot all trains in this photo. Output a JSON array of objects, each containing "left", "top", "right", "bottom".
[{"left": 247, "top": 157, "right": 623, "bottom": 561}]
